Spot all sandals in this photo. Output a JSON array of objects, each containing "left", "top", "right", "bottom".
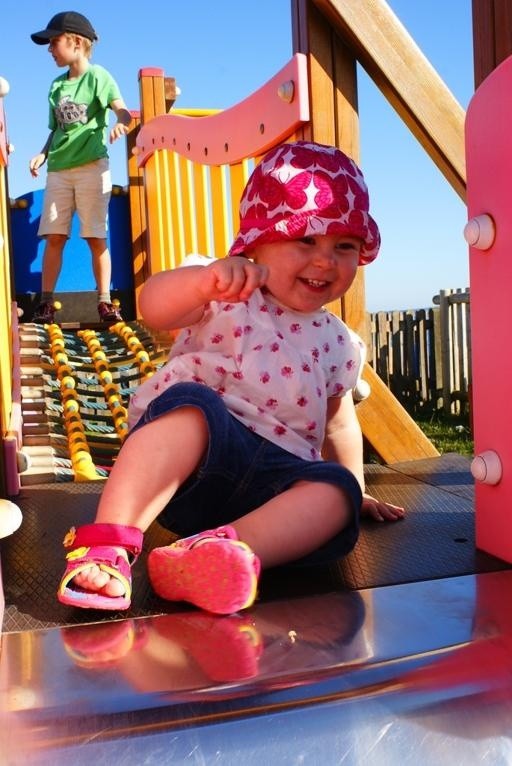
[
  {"left": 57, "top": 523, "right": 144, "bottom": 611},
  {"left": 148, "top": 524, "right": 260, "bottom": 616}
]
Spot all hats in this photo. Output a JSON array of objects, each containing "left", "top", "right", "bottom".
[
  {"left": 227, "top": 141, "right": 380, "bottom": 265},
  {"left": 31, "top": 11, "right": 96, "bottom": 45}
]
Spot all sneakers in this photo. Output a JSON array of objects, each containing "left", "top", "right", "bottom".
[
  {"left": 97, "top": 302, "right": 124, "bottom": 321},
  {"left": 30, "top": 303, "right": 56, "bottom": 323}
]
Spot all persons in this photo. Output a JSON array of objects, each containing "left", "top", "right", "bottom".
[
  {"left": 57, "top": 142, "right": 405, "bottom": 614},
  {"left": 30, "top": 11, "right": 124, "bottom": 323}
]
